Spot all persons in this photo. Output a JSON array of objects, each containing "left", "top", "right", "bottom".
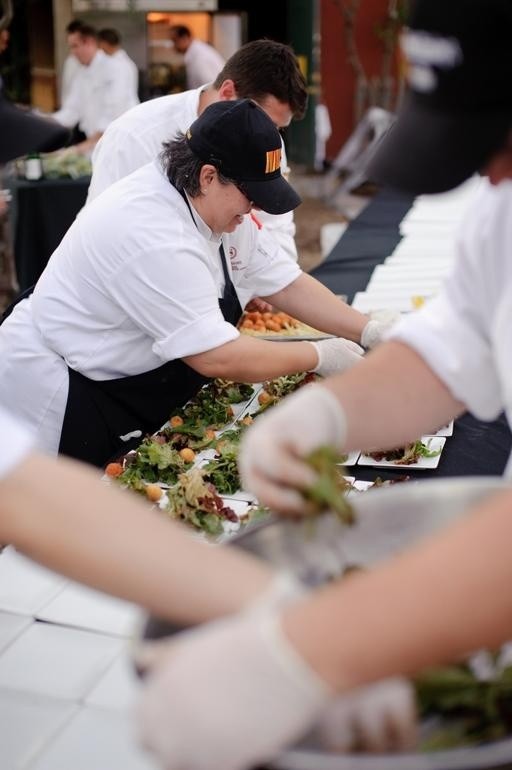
[
  {"left": 44, "top": 17, "right": 141, "bottom": 155},
  {"left": 131, "top": 0, "right": 512, "bottom": 769},
  {"left": 0, "top": 410, "right": 419, "bottom": 759},
  {"left": 0, "top": 1, "right": 88, "bottom": 166},
  {"left": 0, "top": 99, "right": 406, "bottom": 478},
  {"left": 169, "top": 24, "right": 226, "bottom": 89},
  {"left": 83, "top": 39, "right": 309, "bottom": 262}
]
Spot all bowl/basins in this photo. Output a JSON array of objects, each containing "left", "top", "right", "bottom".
[{"left": 136, "top": 476, "right": 512, "bottom": 766}]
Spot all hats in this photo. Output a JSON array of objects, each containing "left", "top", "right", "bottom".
[
  {"left": 345, "top": 2, "right": 512, "bottom": 195},
  {"left": 186, "top": 98, "right": 302, "bottom": 214}
]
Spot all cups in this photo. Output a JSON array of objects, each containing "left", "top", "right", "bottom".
[{"left": 14, "top": 151, "right": 46, "bottom": 185}]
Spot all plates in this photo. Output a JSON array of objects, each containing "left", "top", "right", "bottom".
[
  {"left": 1, "top": 546, "right": 136, "bottom": 767},
  {"left": 84, "top": 294, "right": 455, "bottom": 546}
]
